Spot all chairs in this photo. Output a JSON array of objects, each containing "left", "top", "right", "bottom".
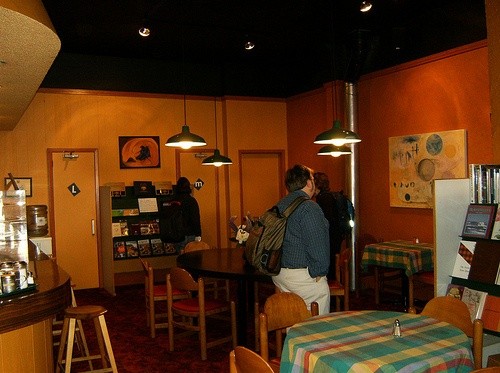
[
  {"left": 138, "top": 248, "right": 349, "bottom": 373},
  {"left": 408, "top": 296, "right": 483, "bottom": 370}
]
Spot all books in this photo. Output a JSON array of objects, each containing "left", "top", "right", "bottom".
[{"left": 446, "top": 164, "right": 500, "bottom": 334}]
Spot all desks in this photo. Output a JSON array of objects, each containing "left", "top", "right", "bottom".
[
  {"left": 176, "top": 247, "right": 337, "bottom": 350},
  {"left": 280, "top": 308, "right": 475, "bottom": 373},
  {"left": 360, "top": 240, "right": 435, "bottom": 311}
]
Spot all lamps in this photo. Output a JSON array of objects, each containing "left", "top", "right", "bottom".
[
  {"left": 313, "top": 41, "right": 362, "bottom": 157},
  {"left": 201, "top": 91, "right": 232, "bottom": 167},
  {"left": 164, "top": 93, "right": 208, "bottom": 149}
]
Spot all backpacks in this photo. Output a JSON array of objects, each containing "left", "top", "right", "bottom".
[
  {"left": 245, "top": 196, "right": 309, "bottom": 276},
  {"left": 331, "top": 190, "right": 354, "bottom": 234},
  {"left": 159, "top": 199, "right": 185, "bottom": 243}
]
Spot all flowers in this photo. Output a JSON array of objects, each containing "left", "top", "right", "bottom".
[{"left": 227, "top": 214, "right": 250, "bottom": 248}]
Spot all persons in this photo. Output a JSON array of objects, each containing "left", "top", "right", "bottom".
[
  {"left": 313, "top": 172, "right": 355, "bottom": 280},
  {"left": 273, "top": 164, "right": 330, "bottom": 335},
  {"left": 159, "top": 177, "right": 201, "bottom": 297}
]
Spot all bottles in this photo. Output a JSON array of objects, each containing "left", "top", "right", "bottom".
[
  {"left": 394, "top": 323, "right": 401, "bottom": 338},
  {"left": 27, "top": 204, "right": 49, "bottom": 237},
  {"left": 0, "top": 260, "right": 35, "bottom": 295},
  {"left": 393, "top": 319, "right": 399, "bottom": 332}
]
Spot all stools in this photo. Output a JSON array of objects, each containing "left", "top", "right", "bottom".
[{"left": 50, "top": 282, "right": 119, "bottom": 373}]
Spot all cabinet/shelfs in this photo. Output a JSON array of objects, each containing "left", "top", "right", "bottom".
[{"left": 98, "top": 185, "right": 174, "bottom": 296}]
